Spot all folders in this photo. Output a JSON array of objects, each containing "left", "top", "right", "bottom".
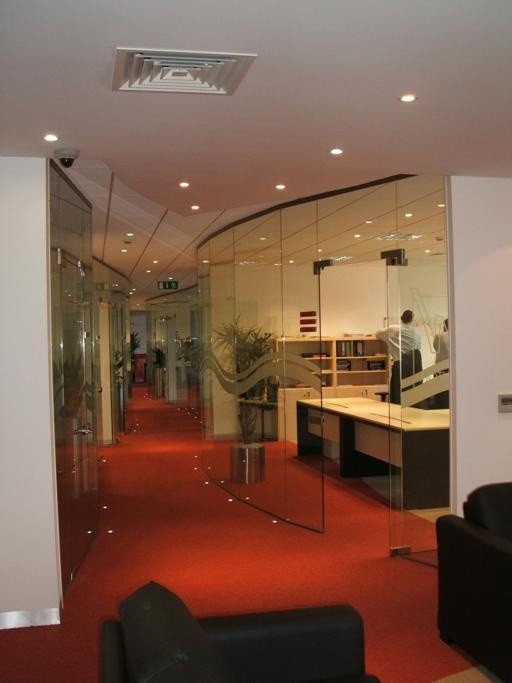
[{"left": 337, "top": 341, "right": 364, "bottom": 357}]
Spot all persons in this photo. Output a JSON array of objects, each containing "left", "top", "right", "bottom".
[
  {"left": 378, "top": 308, "right": 421, "bottom": 401},
  {"left": 433, "top": 315, "right": 452, "bottom": 381}
]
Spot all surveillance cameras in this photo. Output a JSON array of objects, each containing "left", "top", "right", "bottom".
[{"left": 58, "top": 155, "right": 75, "bottom": 168}]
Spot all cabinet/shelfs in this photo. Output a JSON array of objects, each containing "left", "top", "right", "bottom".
[{"left": 276, "top": 337, "right": 392, "bottom": 443}]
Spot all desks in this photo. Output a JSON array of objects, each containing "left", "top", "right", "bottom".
[{"left": 297, "top": 396, "right": 450, "bottom": 508}]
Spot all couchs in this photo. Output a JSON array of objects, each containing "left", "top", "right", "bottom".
[
  {"left": 98, "top": 578, "right": 382, "bottom": 682},
  {"left": 434, "top": 480, "right": 512, "bottom": 682}
]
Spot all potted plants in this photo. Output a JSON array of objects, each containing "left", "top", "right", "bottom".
[
  {"left": 219, "top": 314, "right": 280, "bottom": 485},
  {"left": 53, "top": 354, "right": 95, "bottom": 502}
]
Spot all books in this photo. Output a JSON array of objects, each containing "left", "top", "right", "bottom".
[
  {"left": 302, "top": 352, "right": 329, "bottom": 357},
  {"left": 337, "top": 341, "right": 342, "bottom": 356},
  {"left": 357, "top": 342, "right": 363, "bottom": 356},
  {"left": 347, "top": 341, "right": 350, "bottom": 356},
  {"left": 343, "top": 340, "right": 346, "bottom": 356}
]
